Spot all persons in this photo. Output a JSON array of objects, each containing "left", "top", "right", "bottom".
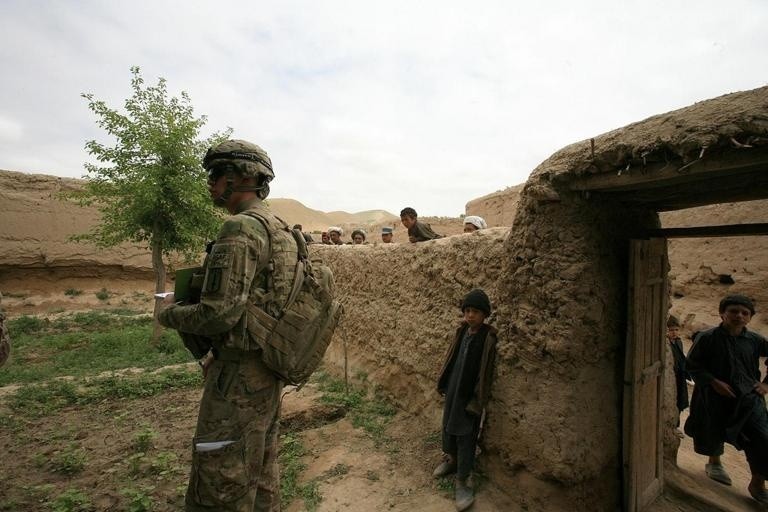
[
  {"left": 399, "top": 207, "right": 444, "bottom": 243},
  {"left": 0, "top": 313, "right": 11, "bottom": 369},
  {"left": 157, "top": 139, "right": 308, "bottom": 512},
  {"left": 321, "top": 232, "right": 333, "bottom": 245},
  {"left": 462, "top": 215, "right": 487, "bottom": 234},
  {"left": 380, "top": 227, "right": 395, "bottom": 243},
  {"left": 328, "top": 227, "right": 346, "bottom": 245},
  {"left": 348, "top": 230, "right": 369, "bottom": 244},
  {"left": 292, "top": 224, "right": 313, "bottom": 243},
  {"left": 430, "top": 287, "right": 497, "bottom": 510},
  {"left": 683, "top": 294, "right": 768, "bottom": 502},
  {"left": 667, "top": 314, "right": 690, "bottom": 438}
]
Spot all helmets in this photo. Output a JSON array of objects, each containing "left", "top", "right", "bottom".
[{"left": 202, "top": 139, "right": 275, "bottom": 182}]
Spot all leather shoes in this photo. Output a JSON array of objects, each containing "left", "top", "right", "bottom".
[
  {"left": 747, "top": 485, "right": 767, "bottom": 505},
  {"left": 433, "top": 459, "right": 456, "bottom": 477},
  {"left": 704, "top": 464, "right": 732, "bottom": 486},
  {"left": 455, "top": 487, "right": 474, "bottom": 512}
]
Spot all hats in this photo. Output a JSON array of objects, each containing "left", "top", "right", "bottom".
[
  {"left": 461, "top": 289, "right": 490, "bottom": 317},
  {"left": 381, "top": 226, "right": 392, "bottom": 235}
]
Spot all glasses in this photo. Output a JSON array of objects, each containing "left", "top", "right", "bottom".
[{"left": 208, "top": 166, "right": 226, "bottom": 181}]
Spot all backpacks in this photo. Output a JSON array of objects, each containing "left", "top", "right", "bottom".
[{"left": 241, "top": 208, "right": 343, "bottom": 403}]
